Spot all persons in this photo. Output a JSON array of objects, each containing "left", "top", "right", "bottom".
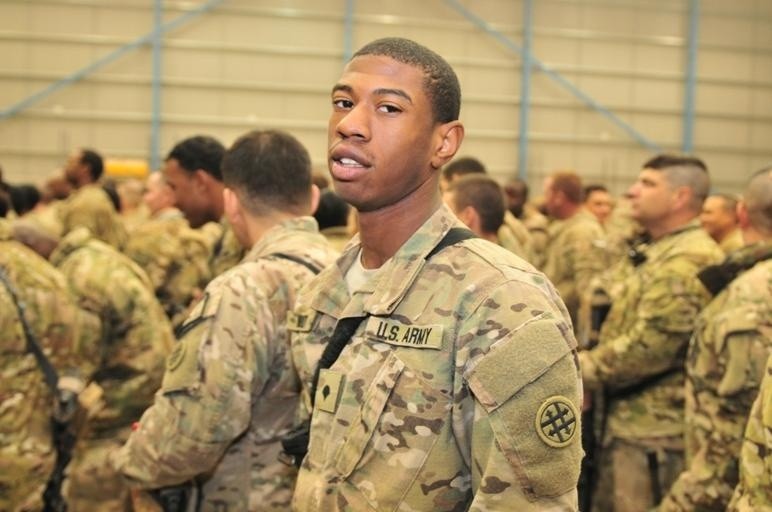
[
  {"left": 443, "top": 154, "right": 772, "bottom": 511},
  {"left": 0, "top": 130, "right": 356, "bottom": 511},
  {"left": 292, "top": 36, "right": 582, "bottom": 511}
]
